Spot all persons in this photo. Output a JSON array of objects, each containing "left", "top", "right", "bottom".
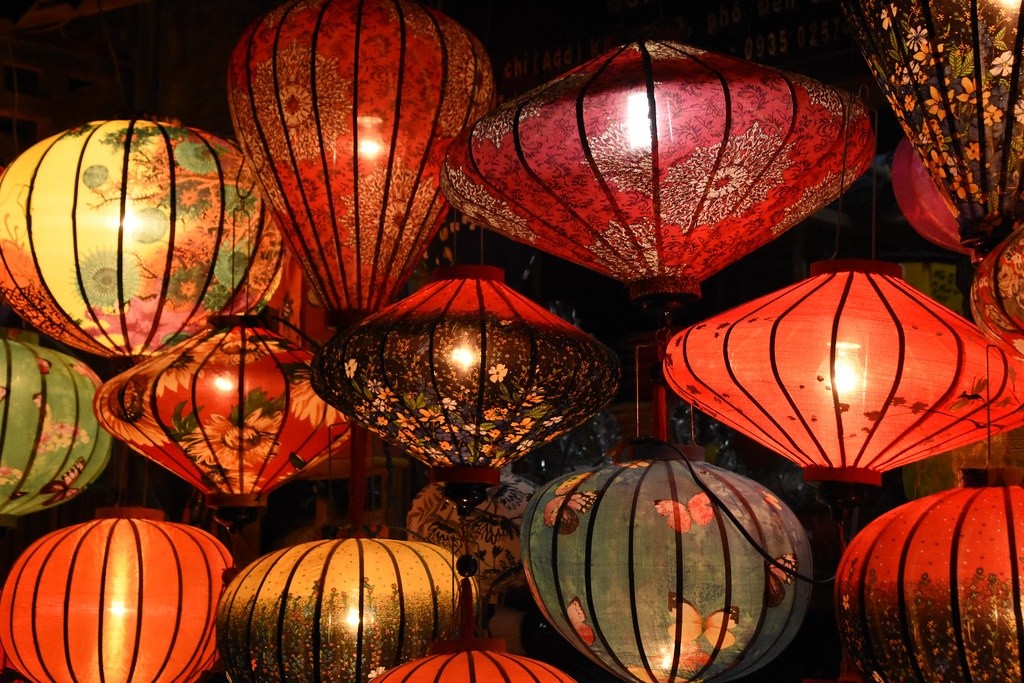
[{"left": 0, "top": 0, "right": 1024, "bottom": 683}]
